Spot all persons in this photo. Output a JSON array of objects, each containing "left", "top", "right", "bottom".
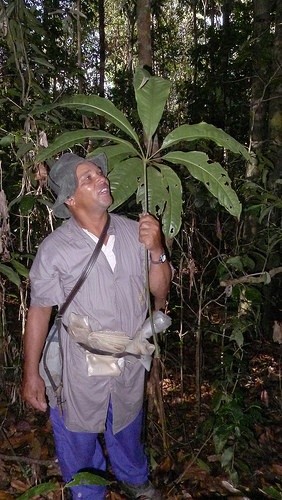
[{"left": 21, "top": 153, "right": 173, "bottom": 500}]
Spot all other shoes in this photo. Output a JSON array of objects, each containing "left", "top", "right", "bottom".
[{"left": 119, "top": 477, "right": 161, "bottom": 500}]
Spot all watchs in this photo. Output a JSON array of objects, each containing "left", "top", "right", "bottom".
[{"left": 149, "top": 247, "right": 166, "bottom": 265}]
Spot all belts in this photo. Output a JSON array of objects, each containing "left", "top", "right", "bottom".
[{"left": 62, "top": 322, "right": 140, "bottom": 360}]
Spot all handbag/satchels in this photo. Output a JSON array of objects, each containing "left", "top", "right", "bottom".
[{"left": 39, "top": 319, "right": 64, "bottom": 408}]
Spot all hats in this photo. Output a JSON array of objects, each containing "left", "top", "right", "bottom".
[{"left": 48, "top": 152, "right": 108, "bottom": 218}]
